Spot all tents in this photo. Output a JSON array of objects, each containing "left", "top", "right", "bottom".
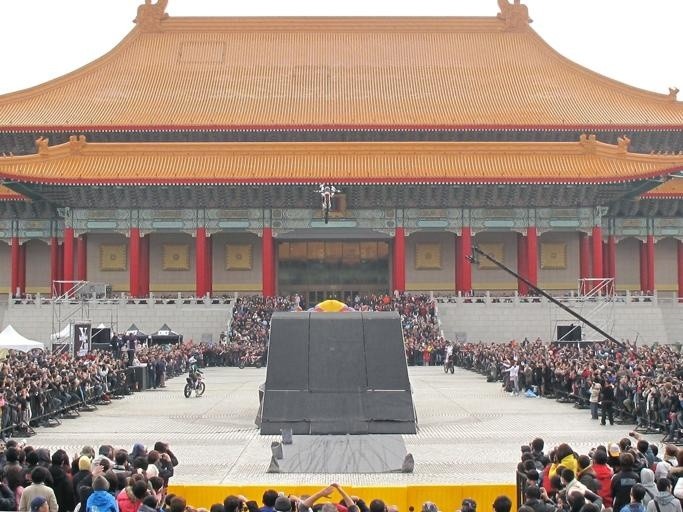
[
  {"left": 50, "top": 323, "right": 183, "bottom": 345},
  {"left": 0, "top": 324, "right": 44, "bottom": 353}
]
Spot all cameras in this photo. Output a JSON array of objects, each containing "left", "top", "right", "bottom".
[{"left": 629, "top": 433, "right": 635, "bottom": 436}]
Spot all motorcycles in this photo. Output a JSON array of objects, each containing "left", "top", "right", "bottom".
[{"left": 312, "top": 184, "right": 342, "bottom": 225}]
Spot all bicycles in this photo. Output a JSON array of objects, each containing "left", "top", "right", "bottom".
[
  {"left": 184, "top": 372, "right": 207, "bottom": 399},
  {"left": 444, "top": 356, "right": 454, "bottom": 374}
]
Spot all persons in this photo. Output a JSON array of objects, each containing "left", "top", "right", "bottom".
[
  {"left": 493, "top": 495, "right": 512, "bottom": 512},
  {"left": 0, "top": 439, "right": 390, "bottom": 512},
  {"left": 461, "top": 497, "right": 476, "bottom": 512},
  {"left": 133, "top": 341, "right": 268, "bottom": 387},
  {"left": 444, "top": 337, "right": 682, "bottom": 445},
  {"left": 219, "top": 292, "right": 307, "bottom": 347},
  {"left": 342, "top": 289, "right": 445, "bottom": 366},
  {"left": 516, "top": 431, "right": 682, "bottom": 511},
  {"left": 422, "top": 501, "right": 438, "bottom": 511},
  {"left": 0, "top": 347, "right": 132, "bottom": 439}
]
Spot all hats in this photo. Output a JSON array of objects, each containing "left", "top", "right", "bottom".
[
  {"left": 29, "top": 496, "right": 48, "bottom": 511},
  {"left": 132, "top": 443, "right": 145, "bottom": 453},
  {"left": 421, "top": 502, "right": 438, "bottom": 512},
  {"left": 92, "top": 475, "right": 111, "bottom": 491},
  {"left": 80, "top": 445, "right": 95, "bottom": 457},
  {"left": 607, "top": 443, "right": 621, "bottom": 458},
  {"left": 274, "top": 496, "right": 292, "bottom": 511},
  {"left": 99, "top": 445, "right": 114, "bottom": 457}
]
